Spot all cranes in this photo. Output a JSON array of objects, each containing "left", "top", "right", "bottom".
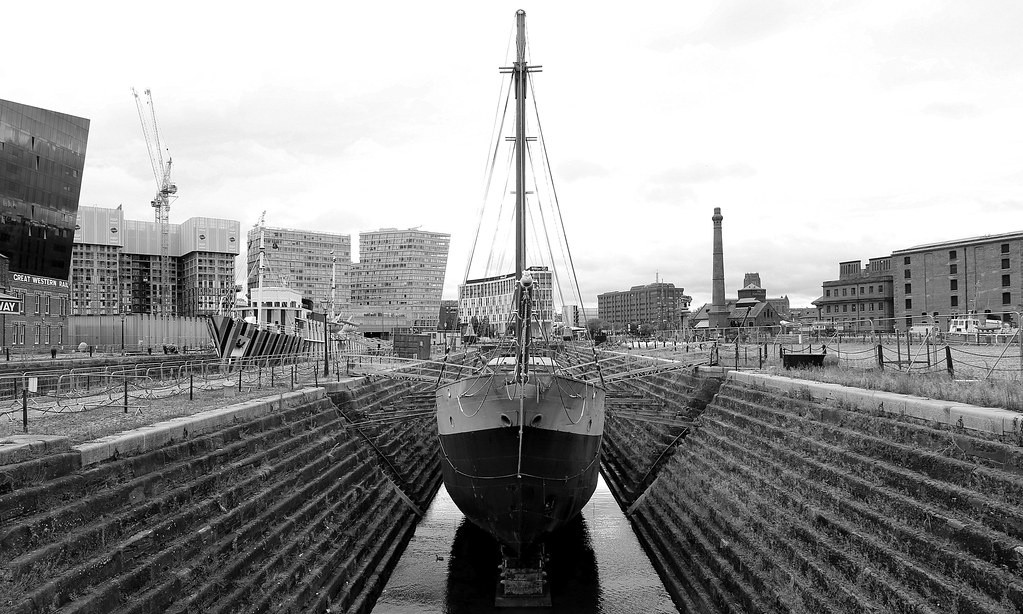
[{"left": 129, "top": 85, "right": 179, "bottom": 356}]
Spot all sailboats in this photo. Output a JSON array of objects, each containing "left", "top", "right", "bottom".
[
  {"left": 205, "top": 210, "right": 368, "bottom": 373},
  {"left": 434, "top": 9, "right": 608, "bottom": 562}
]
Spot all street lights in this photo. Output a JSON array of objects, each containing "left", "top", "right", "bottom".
[
  {"left": 444, "top": 322, "right": 448, "bottom": 351},
  {"left": 320, "top": 295, "right": 331, "bottom": 378},
  {"left": 120, "top": 313, "right": 126, "bottom": 356}
]
